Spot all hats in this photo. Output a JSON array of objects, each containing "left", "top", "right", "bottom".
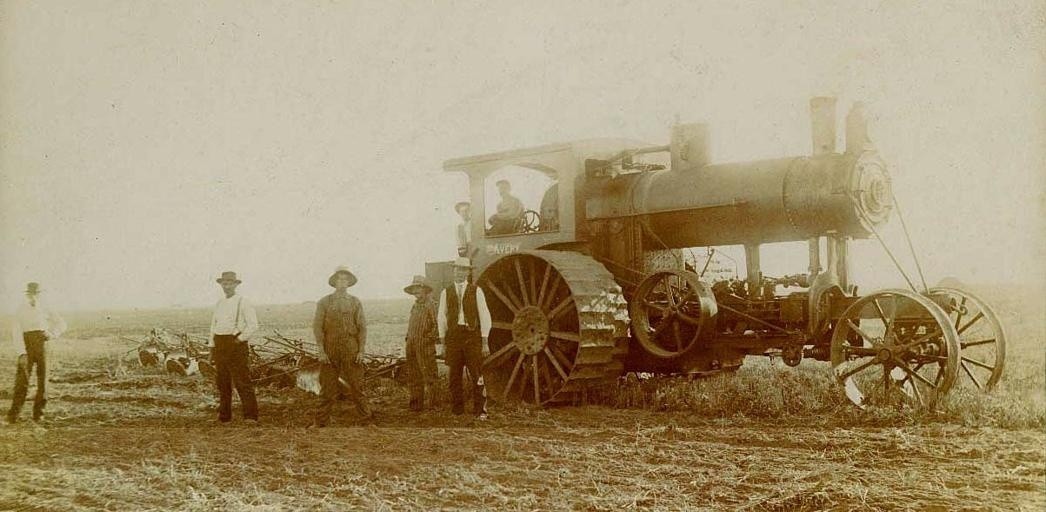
[
  {"left": 495, "top": 178, "right": 512, "bottom": 191},
  {"left": 400, "top": 271, "right": 437, "bottom": 295},
  {"left": 453, "top": 199, "right": 471, "bottom": 215},
  {"left": 452, "top": 257, "right": 475, "bottom": 270},
  {"left": 327, "top": 264, "right": 358, "bottom": 289},
  {"left": 216, "top": 268, "right": 242, "bottom": 287},
  {"left": 24, "top": 281, "right": 45, "bottom": 295}
]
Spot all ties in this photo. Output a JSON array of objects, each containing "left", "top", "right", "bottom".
[{"left": 457, "top": 283, "right": 463, "bottom": 314}]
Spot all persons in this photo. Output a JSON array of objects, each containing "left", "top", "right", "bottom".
[
  {"left": 308, "top": 264, "right": 376, "bottom": 429},
  {"left": 3, "top": 280, "right": 66, "bottom": 423},
  {"left": 437, "top": 256, "right": 492, "bottom": 422},
  {"left": 403, "top": 275, "right": 442, "bottom": 410},
  {"left": 488, "top": 179, "right": 525, "bottom": 236},
  {"left": 206, "top": 271, "right": 260, "bottom": 423},
  {"left": 454, "top": 201, "right": 473, "bottom": 256}
]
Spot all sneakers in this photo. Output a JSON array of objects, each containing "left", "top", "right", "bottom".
[{"left": 213, "top": 419, "right": 379, "bottom": 432}]
